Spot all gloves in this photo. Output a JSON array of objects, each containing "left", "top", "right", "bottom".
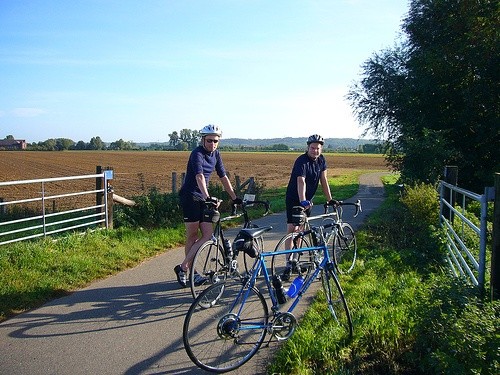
[
  {"left": 232, "top": 198, "right": 243, "bottom": 209},
  {"left": 206, "top": 196, "right": 221, "bottom": 210}
]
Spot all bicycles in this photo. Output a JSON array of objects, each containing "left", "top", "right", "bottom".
[
  {"left": 181, "top": 226, "right": 356, "bottom": 372},
  {"left": 272, "top": 200, "right": 364, "bottom": 294},
  {"left": 188, "top": 197, "right": 271, "bottom": 306}
]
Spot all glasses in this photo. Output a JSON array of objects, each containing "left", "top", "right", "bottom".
[{"left": 205, "top": 138, "right": 218, "bottom": 143}]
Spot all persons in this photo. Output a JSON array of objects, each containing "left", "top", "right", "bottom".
[
  {"left": 281, "top": 134, "right": 338, "bottom": 282},
  {"left": 174, "top": 124, "right": 242, "bottom": 287}
]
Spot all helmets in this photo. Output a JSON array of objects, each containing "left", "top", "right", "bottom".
[
  {"left": 199, "top": 123, "right": 222, "bottom": 137},
  {"left": 307, "top": 134, "right": 324, "bottom": 145}
]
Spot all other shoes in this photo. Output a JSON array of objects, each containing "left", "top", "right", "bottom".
[
  {"left": 292, "top": 266, "right": 307, "bottom": 274},
  {"left": 282, "top": 267, "right": 291, "bottom": 281}
]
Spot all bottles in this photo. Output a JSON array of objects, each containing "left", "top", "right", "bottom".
[
  {"left": 287, "top": 274, "right": 306, "bottom": 298},
  {"left": 315, "top": 230, "right": 321, "bottom": 247},
  {"left": 224, "top": 238, "right": 233, "bottom": 259},
  {"left": 272, "top": 274, "right": 287, "bottom": 304}
]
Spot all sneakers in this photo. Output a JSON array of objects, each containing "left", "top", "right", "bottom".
[
  {"left": 189, "top": 272, "right": 207, "bottom": 285},
  {"left": 174, "top": 264, "right": 187, "bottom": 286}
]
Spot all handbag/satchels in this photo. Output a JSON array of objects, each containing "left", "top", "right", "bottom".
[{"left": 232, "top": 220, "right": 258, "bottom": 258}]
